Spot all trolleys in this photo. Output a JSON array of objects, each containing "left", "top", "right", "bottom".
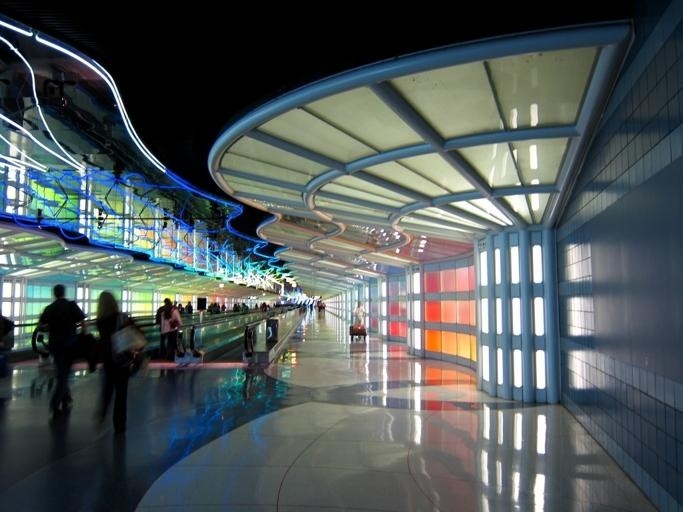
[{"left": 350, "top": 325, "right": 367, "bottom": 342}]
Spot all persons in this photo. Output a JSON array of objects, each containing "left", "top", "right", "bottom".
[
  {"left": 154, "top": 296, "right": 328, "bottom": 361},
  {"left": 46, "top": 406, "right": 76, "bottom": 460},
  {"left": 354, "top": 301, "right": 368, "bottom": 341},
  {"left": 36, "top": 283, "right": 87, "bottom": 414},
  {"left": 91, "top": 413, "right": 128, "bottom": 482},
  {"left": 93, "top": 289, "right": 144, "bottom": 434}
]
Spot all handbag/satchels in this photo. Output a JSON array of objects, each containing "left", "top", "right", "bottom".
[
  {"left": 109, "top": 325, "right": 148, "bottom": 355},
  {"left": 169, "top": 319, "right": 180, "bottom": 329}
]
